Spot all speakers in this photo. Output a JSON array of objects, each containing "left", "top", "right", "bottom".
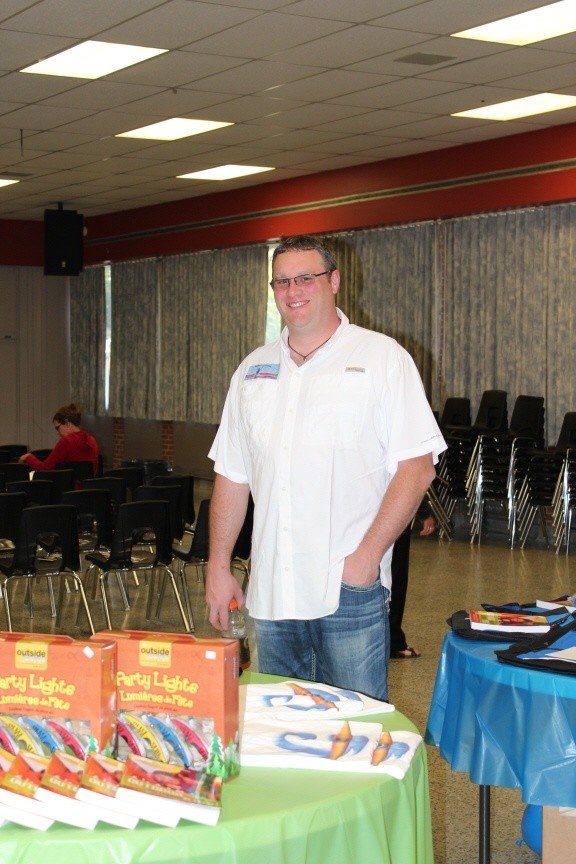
[{"left": 43, "top": 208, "right": 84, "bottom": 275}]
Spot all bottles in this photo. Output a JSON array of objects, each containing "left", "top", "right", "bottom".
[{"left": 221, "top": 600, "right": 252, "bottom": 671}]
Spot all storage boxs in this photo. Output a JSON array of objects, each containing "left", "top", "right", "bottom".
[
  {"left": 542, "top": 805, "right": 576, "bottom": 864},
  {"left": 0, "top": 628, "right": 241, "bottom": 746}
]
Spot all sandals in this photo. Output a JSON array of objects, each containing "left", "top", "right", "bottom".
[{"left": 391, "top": 649, "right": 420, "bottom": 658}]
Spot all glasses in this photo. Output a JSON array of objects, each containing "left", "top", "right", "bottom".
[
  {"left": 55, "top": 424, "right": 61, "bottom": 430},
  {"left": 270, "top": 268, "right": 336, "bottom": 290}
]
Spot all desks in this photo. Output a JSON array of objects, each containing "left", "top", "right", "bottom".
[
  {"left": 0, "top": 667, "right": 434, "bottom": 864},
  {"left": 423, "top": 629, "right": 576, "bottom": 864}
]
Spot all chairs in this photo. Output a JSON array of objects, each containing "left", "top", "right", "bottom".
[
  {"left": 408, "top": 389, "right": 576, "bottom": 556},
  {"left": 0, "top": 444, "right": 255, "bottom": 633}
]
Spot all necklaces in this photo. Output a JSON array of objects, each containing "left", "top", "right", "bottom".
[{"left": 288, "top": 319, "right": 342, "bottom": 361}]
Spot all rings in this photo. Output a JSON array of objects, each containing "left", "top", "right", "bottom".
[{"left": 430, "top": 528, "right": 432, "bottom": 530}]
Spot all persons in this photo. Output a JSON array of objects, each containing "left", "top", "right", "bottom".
[
  {"left": 205, "top": 234, "right": 435, "bottom": 701},
  {"left": 19, "top": 402, "right": 99, "bottom": 491}
]
